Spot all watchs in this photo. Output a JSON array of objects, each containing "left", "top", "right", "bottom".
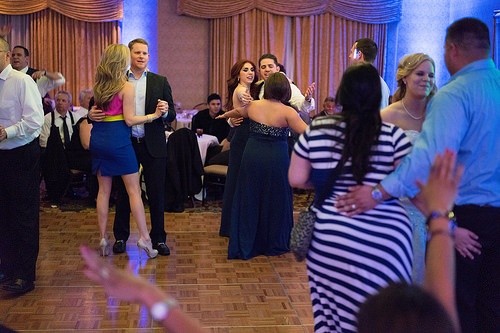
[{"left": 371, "top": 185, "right": 385, "bottom": 204}]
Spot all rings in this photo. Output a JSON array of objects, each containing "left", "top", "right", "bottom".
[{"left": 351, "top": 204, "right": 356, "bottom": 208}]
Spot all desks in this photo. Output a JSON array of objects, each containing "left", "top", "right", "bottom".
[{"left": 139, "top": 130, "right": 220, "bottom": 202}]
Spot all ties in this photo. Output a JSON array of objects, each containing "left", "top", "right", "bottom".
[{"left": 60, "top": 115, "right": 70, "bottom": 148}]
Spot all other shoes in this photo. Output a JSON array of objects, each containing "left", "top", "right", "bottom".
[{"left": 0, "top": 275, "right": 35, "bottom": 299}]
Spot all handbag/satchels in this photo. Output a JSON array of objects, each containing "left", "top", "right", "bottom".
[{"left": 290, "top": 211, "right": 317, "bottom": 263}]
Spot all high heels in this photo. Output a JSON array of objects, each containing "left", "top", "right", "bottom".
[
  {"left": 98, "top": 238, "right": 109, "bottom": 259},
  {"left": 137, "top": 239, "right": 158, "bottom": 258}
]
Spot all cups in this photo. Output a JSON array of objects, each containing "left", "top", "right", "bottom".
[{"left": 182, "top": 110, "right": 198, "bottom": 119}]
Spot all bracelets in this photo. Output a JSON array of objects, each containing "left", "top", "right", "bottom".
[
  {"left": 42, "top": 70, "right": 47, "bottom": 76},
  {"left": 426, "top": 210, "right": 451, "bottom": 225},
  {"left": 149, "top": 295, "right": 177, "bottom": 323},
  {"left": 428, "top": 228, "right": 455, "bottom": 238},
  {"left": 146, "top": 115, "right": 153, "bottom": 123}
]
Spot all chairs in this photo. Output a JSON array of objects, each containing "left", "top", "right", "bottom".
[
  {"left": 56, "top": 142, "right": 93, "bottom": 210},
  {"left": 186, "top": 103, "right": 208, "bottom": 119},
  {"left": 202, "top": 163, "right": 230, "bottom": 208},
  {"left": 139, "top": 128, "right": 207, "bottom": 212}
]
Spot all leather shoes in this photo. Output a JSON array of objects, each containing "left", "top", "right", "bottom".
[
  {"left": 113, "top": 239, "right": 126, "bottom": 253},
  {"left": 151, "top": 239, "right": 170, "bottom": 256}
]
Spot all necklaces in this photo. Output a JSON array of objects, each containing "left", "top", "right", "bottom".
[{"left": 401, "top": 98, "right": 423, "bottom": 120}]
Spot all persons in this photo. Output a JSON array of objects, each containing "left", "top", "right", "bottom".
[{"left": 0, "top": 17, "right": 500, "bottom": 333}]
